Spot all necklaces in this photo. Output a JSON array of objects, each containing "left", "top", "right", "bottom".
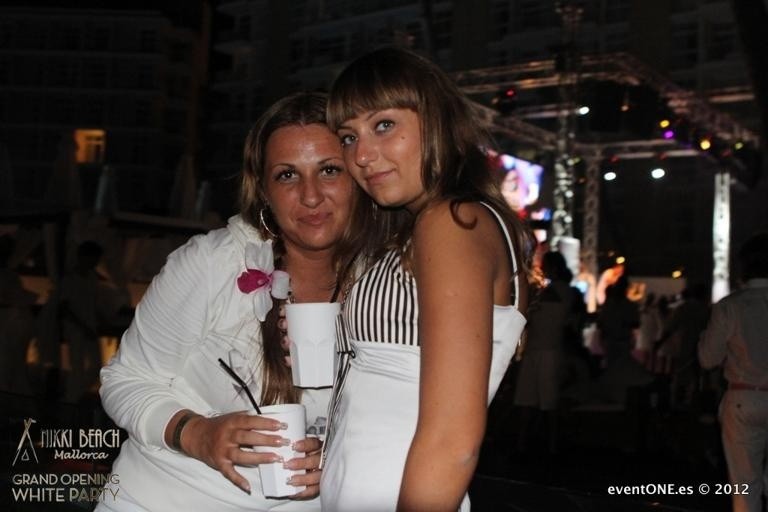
[{"left": 338, "top": 269, "right": 354, "bottom": 309}]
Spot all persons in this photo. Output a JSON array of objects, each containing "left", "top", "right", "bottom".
[
  {"left": 506, "top": 232, "right": 768, "bottom": 510},
  {"left": 88, "top": 86, "right": 380, "bottom": 512},
  {"left": 274, "top": 44, "right": 536, "bottom": 512},
  {"left": 36, "top": 230, "right": 127, "bottom": 407},
  {"left": 1, "top": 232, "right": 42, "bottom": 398}
]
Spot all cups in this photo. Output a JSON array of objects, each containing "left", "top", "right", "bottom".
[
  {"left": 285, "top": 303, "right": 341, "bottom": 390},
  {"left": 249, "top": 404, "right": 308, "bottom": 499}
]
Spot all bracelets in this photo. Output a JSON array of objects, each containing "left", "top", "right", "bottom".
[{"left": 172, "top": 412, "right": 203, "bottom": 451}]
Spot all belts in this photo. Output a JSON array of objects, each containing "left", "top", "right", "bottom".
[{"left": 728, "top": 383, "right": 768, "bottom": 392}]
